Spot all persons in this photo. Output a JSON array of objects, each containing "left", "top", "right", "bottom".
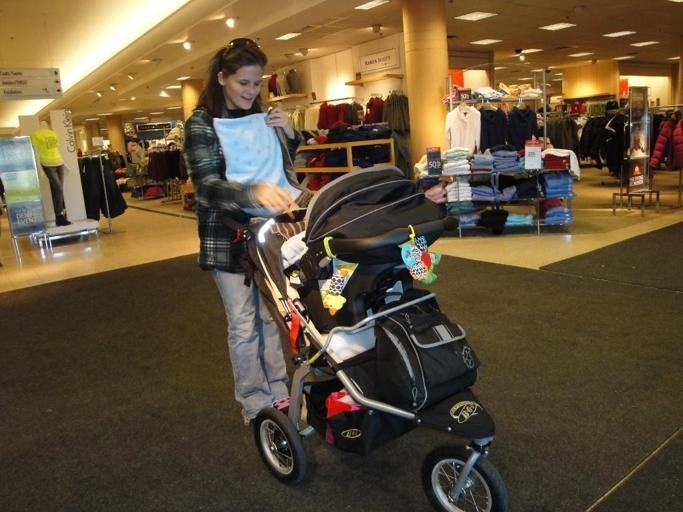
[
  {"left": 32, "top": 118, "right": 73, "bottom": 228},
  {"left": 182, "top": 36, "right": 309, "bottom": 430}
]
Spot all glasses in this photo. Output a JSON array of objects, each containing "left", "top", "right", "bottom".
[{"left": 227, "top": 38, "right": 254, "bottom": 55}]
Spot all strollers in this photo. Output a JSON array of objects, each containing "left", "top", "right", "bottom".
[{"left": 224, "top": 163, "right": 510, "bottom": 512}]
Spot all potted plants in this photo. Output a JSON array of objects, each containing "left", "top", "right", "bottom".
[{"left": 125, "top": 163, "right": 138, "bottom": 187}]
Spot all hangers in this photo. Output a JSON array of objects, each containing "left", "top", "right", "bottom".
[
  {"left": 458, "top": 95, "right": 473, "bottom": 116},
  {"left": 514, "top": 97, "right": 528, "bottom": 110},
  {"left": 478, "top": 98, "right": 498, "bottom": 110},
  {"left": 368, "top": 88, "right": 404, "bottom": 99}
]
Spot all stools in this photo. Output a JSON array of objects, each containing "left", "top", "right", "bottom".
[{"left": 27, "top": 214, "right": 102, "bottom": 251}]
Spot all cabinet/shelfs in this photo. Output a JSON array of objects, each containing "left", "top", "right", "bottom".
[
  {"left": 291, "top": 137, "right": 395, "bottom": 197},
  {"left": 417, "top": 170, "right": 573, "bottom": 240}
]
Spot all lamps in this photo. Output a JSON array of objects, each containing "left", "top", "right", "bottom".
[
  {"left": 300, "top": 48, "right": 309, "bottom": 56},
  {"left": 97, "top": 73, "right": 134, "bottom": 97},
  {"left": 370, "top": 24, "right": 382, "bottom": 31}
]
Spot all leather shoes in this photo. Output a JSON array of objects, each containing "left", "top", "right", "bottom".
[{"left": 56, "top": 216, "right": 71, "bottom": 225}]
